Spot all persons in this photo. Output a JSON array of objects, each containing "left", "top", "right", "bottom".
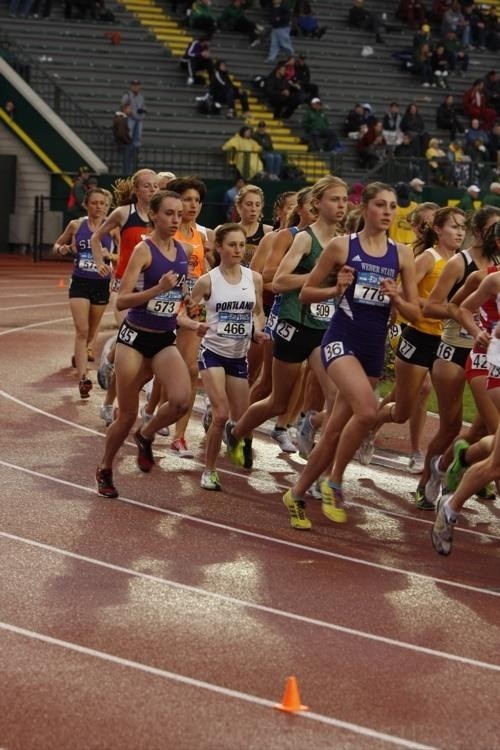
[{"left": 8, "top": 0, "right": 500, "bottom": 556}]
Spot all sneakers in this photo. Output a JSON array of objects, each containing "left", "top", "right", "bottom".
[
  {"left": 72, "top": 334, "right": 118, "bottom": 427},
  {"left": 96, "top": 466, "right": 118, "bottom": 498},
  {"left": 133, "top": 391, "right": 194, "bottom": 473},
  {"left": 358, "top": 429, "right": 379, "bottom": 466},
  {"left": 408, "top": 438, "right": 498, "bottom": 555},
  {"left": 271, "top": 409, "right": 348, "bottom": 530},
  {"left": 200, "top": 404, "right": 253, "bottom": 490}
]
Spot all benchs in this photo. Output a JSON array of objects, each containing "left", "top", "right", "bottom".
[{"left": 0, "top": 0, "right": 500, "bottom": 189}]
